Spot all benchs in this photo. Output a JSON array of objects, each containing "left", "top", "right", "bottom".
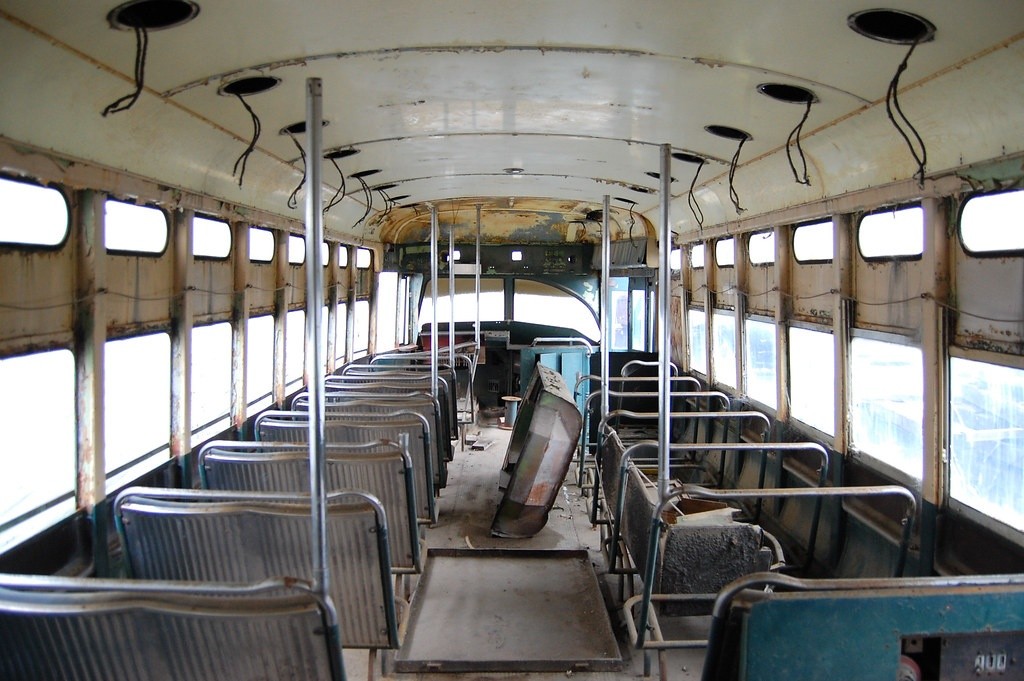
[
  {"left": 699, "top": 573, "right": 1024, "bottom": 680},
  {"left": 0, "top": 352, "right": 477, "bottom": 681}
]
